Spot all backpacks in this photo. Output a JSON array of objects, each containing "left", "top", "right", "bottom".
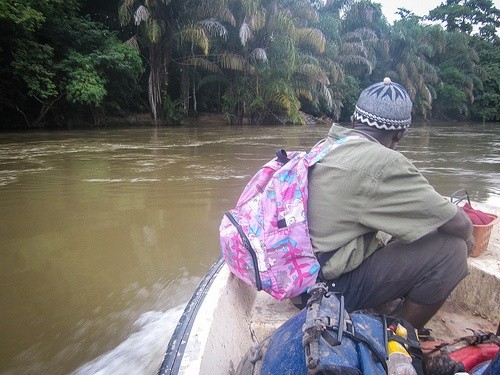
[{"left": 217, "top": 135, "right": 368, "bottom": 301}]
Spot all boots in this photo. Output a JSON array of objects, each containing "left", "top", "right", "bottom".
[{"left": 374, "top": 294, "right": 446, "bottom": 336}]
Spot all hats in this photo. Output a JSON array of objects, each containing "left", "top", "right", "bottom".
[{"left": 352, "top": 78, "right": 413, "bottom": 130}]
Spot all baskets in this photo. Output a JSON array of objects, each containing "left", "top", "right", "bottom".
[{"left": 450, "top": 188, "right": 500, "bottom": 257}]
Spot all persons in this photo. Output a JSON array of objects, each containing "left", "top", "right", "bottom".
[{"left": 288, "top": 77, "right": 477, "bottom": 343}]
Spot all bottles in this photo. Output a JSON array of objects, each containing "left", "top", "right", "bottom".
[{"left": 386, "top": 340, "right": 417, "bottom": 375}]
